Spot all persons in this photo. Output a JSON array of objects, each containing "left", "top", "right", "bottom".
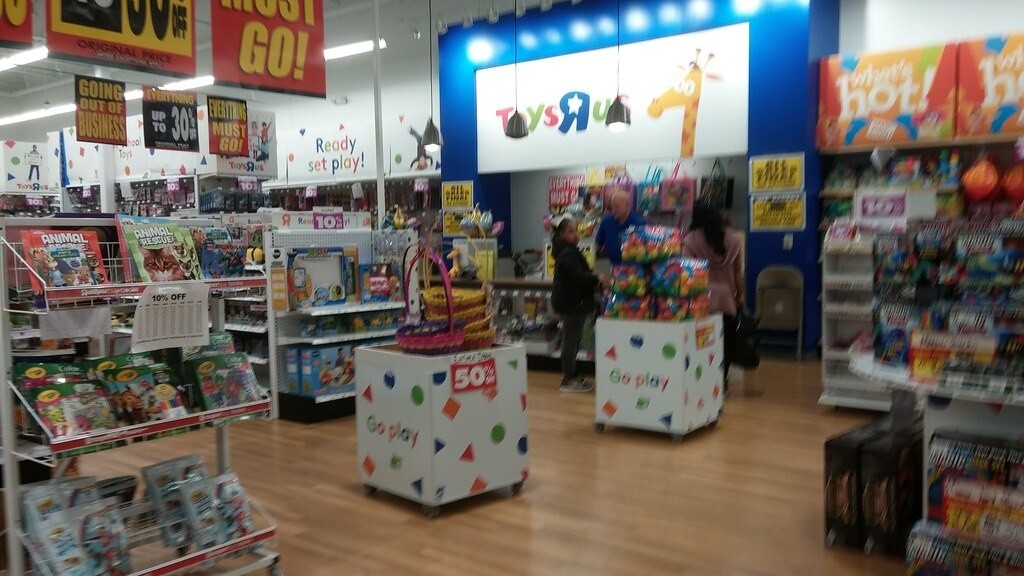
[
  {"left": 682, "top": 197, "right": 746, "bottom": 400},
  {"left": 552, "top": 220, "right": 607, "bottom": 393},
  {"left": 595, "top": 190, "right": 648, "bottom": 273}
]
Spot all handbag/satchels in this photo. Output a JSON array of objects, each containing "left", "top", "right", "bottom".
[
  {"left": 601, "top": 156, "right": 736, "bottom": 216},
  {"left": 730, "top": 303, "right": 762, "bottom": 369}
]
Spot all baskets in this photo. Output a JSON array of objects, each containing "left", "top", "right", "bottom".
[{"left": 396, "top": 213, "right": 497, "bottom": 355}]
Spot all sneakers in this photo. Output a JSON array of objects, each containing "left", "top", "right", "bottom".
[{"left": 559, "top": 376, "right": 594, "bottom": 392}]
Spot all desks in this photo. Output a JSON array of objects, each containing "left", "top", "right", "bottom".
[
  {"left": 354, "top": 343, "right": 530, "bottom": 517},
  {"left": 595, "top": 313, "right": 724, "bottom": 440}
]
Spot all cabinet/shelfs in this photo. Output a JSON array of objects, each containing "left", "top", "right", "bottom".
[
  {"left": 108, "top": 211, "right": 414, "bottom": 418},
  {"left": 817, "top": 188, "right": 937, "bottom": 408},
  {"left": 0, "top": 217, "right": 283, "bottom": 576},
  {"left": 849, "top": 352, "right": 1024, "bottom": 576}
]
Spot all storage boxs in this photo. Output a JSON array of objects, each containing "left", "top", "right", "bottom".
[
  {"left": 824, "top": 220, "right": 1024, "bottom": 557},
  {"left": 816, "top": 41, "right": 957, "bottom": 147},
  {"left": 956, "top": 34, "right": 1024, "bottom": 137}
]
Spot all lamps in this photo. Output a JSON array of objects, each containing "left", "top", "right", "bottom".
[{"left": 409, "top": 0, "right": 552, "bottom": 42}]
